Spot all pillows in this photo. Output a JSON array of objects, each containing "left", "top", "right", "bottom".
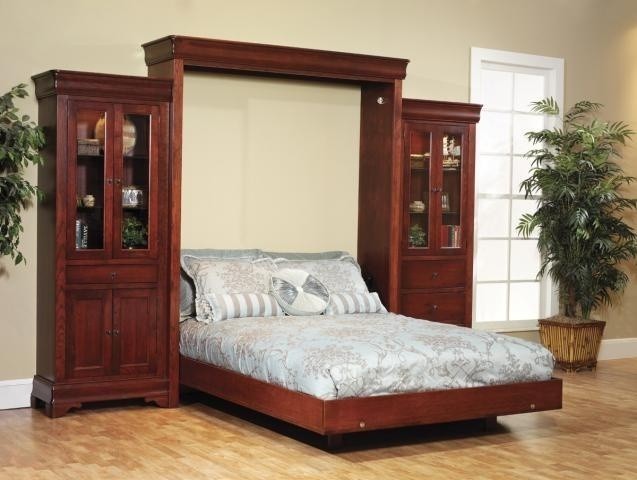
[{"left": 181, "top": 254, "right": 384, "bottom": 323}]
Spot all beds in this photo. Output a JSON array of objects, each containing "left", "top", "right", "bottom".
[{"left": 180, "top": 247, "right": 564, "bottom": 451}]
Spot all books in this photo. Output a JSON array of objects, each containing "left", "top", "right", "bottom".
[
  {"left": 439, "top": 224, "right": 463, "bottom": 249},
  {"left": 75, "top": 220, "right": 82, "bottom": 249},
  {"left": 80, "top": 223, "right": 88, "bottom": 249}
]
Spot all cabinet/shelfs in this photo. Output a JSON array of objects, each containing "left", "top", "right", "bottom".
[
  {"left": 29, "top": 70, "right": 173, "bottom": 419},
  {"left": 397, "top": 96, "right": 483, "bottom": 330}
]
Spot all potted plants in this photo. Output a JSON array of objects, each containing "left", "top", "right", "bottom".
[{"left": 512, "top": 94, "right": 636, "bottom": 374}]
